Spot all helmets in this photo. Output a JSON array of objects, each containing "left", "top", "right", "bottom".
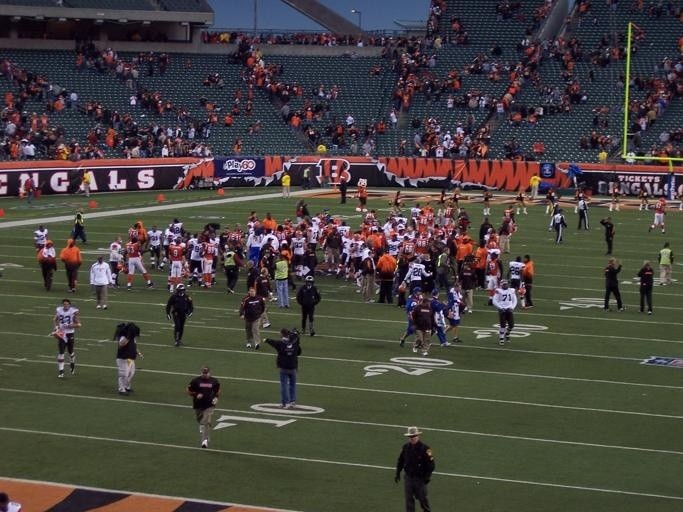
[
  {"left": 173, "top": 283, "right": 186, "bottom": 295},
  {"left": 499, "top": 277, "right": 510, "bottom": 287}
]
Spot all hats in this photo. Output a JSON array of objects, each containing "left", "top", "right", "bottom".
[
  {"left": 199, "top": 366, "right": 211, "bottom": 374},
  {"left": 403, "top": 425, "right": 424, "bottom": 440},
  {"left": 304, "top": 275, "right": 314, "bottom": 288}
]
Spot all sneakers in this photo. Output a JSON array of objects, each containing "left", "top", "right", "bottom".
[
  {"left": 400, "top": 334, "right": 513, "bottom": 357},
  {"left": 173, "top": 336, "right": 186, "bottom": 347},
  {"left": 263, "top": 321, "right": 272, "bottom": 330},
  {"left": 278, "top": 398, "right": 296, "bottom": 411},
  {"left": 88, "top": 261, "right": 166, "bottom": 312},
  {"left": 201, "top": 436, "right": 211, "bottom": 449},
  {"left": 323, "top": 264, "right": 365, "bottom": 286},
  {"left": 602, "top": 302, "right": 658, "bottom": 320},
  {"left": 56, "top": 365, "right": 76, "bottom": 380},
  {"left": 300, "top": 325, "right": 317, "bottom": 338},
  {"left": 187, "top": 272, "right": 237, "bottom": 295},
  {"left": 116, "top": 384, "right": 134, "bottom": 397},
  {"left": 245, "top": 341, "right": 261, "bottom": 351}
]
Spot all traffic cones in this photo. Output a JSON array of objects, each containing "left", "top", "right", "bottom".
[
  {"left": 158, "top": 194, "right": 163, "bottom": 202},
  {"left": 0, "top": 208, "right": 4, "bottom": 217},
  {"left": 217, "top": 188, "right": 224, "bottom": 196},
  {"left": 88, "top": 198, "right": 96, "bottom": 208}
]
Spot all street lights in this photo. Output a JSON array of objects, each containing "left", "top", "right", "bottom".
[{"left": 350, "top": 9, "right": 362, "bottom": 28}]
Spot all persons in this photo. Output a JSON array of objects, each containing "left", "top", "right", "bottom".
[
  {"left": 186, "top": 367, "right": 221, "bottom": 448},
  {"left": 263, "top": 328, "right": 302, "bottom": 409},
  {"left": 5, "top": 0, "right": 682, "bottom": 357},
  {"left": 394, "top": 425, "right": 436, "bottom": 512},
  {"left": 114, "top": 323, "right": 145, "bottom": 395},
  {"left": 53, "top": 298, "right": 81, "bottom": 378}
]
